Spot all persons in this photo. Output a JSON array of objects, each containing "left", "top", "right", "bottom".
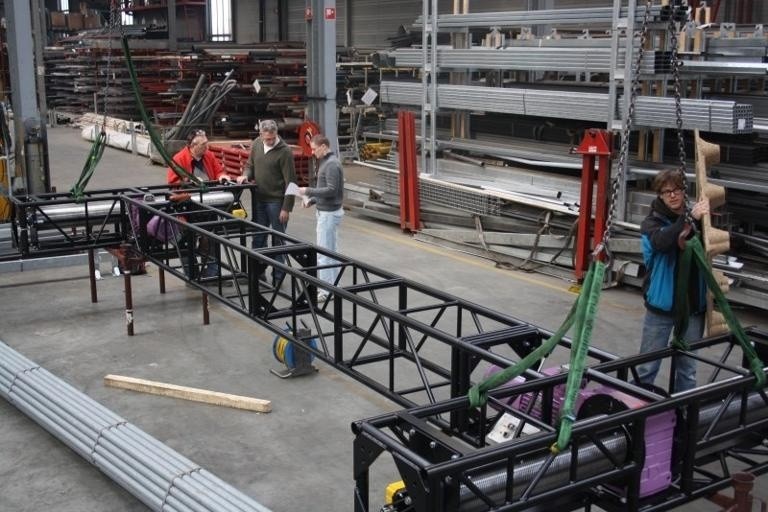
[
  {"left": 167, "top": 127, "right": 233, "bottom": 279},
  {"left": 628, "top": 168, "right": 710, "bottom": 392},
  {"left": 296, "top": 133, "right": 346, "bottom": 304},
  {"left": 236, "top": 118, "right": 297, "bottom": 289}
]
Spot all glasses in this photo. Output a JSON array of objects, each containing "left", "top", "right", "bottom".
[{"left": 662, "top": 188, "right": 681, "bottom": 197}]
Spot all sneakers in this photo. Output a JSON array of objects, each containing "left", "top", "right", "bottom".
[
  {"left": 317, "top": 293, "right": 333, "bottom": 302},
  {"left": 186, "top": 274, "right": 266, "bottom": 289}
]
